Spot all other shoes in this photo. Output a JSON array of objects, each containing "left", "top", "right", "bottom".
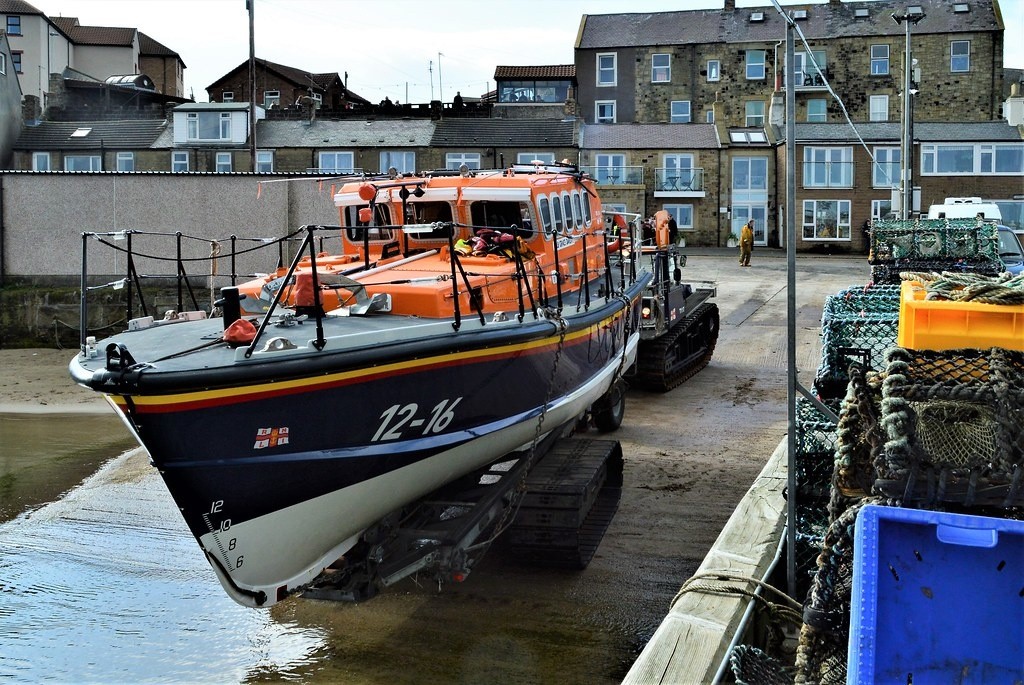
[
  {"left": 739, "top": 262, "right": 743, "bottom": 266},
  {"left": 743, "top": 265, "right": 751, "bottom": 267}
]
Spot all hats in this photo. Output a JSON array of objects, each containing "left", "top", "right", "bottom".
[{"left": 668, "top": 214, "right": 673, "bottom": 217}]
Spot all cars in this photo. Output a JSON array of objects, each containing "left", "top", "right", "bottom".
[{"left": 928, "top": 204, "right": 1024, "bottom": 277}]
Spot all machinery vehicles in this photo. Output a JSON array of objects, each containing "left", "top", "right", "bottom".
[{"left": 621, "top": 249, "right": 720, "bottom": 394}]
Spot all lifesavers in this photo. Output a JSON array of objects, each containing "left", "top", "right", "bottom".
[{"left": 606, "top": 213, "right": 628, "bottom": 252}]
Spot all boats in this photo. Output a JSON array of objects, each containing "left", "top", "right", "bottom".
[{"left": 68, "top": 158, "right": 654, "bottom": 610}]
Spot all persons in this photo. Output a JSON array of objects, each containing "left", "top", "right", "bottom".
[
  {"left": 739, "top": 220, "right": 754, "bottom": 266},
  {"left": 641, "top": 214, "right": 678, "bottom": 246},
  {"left": 864, "top": 219, "right": 870, "bottom": 254}
]
